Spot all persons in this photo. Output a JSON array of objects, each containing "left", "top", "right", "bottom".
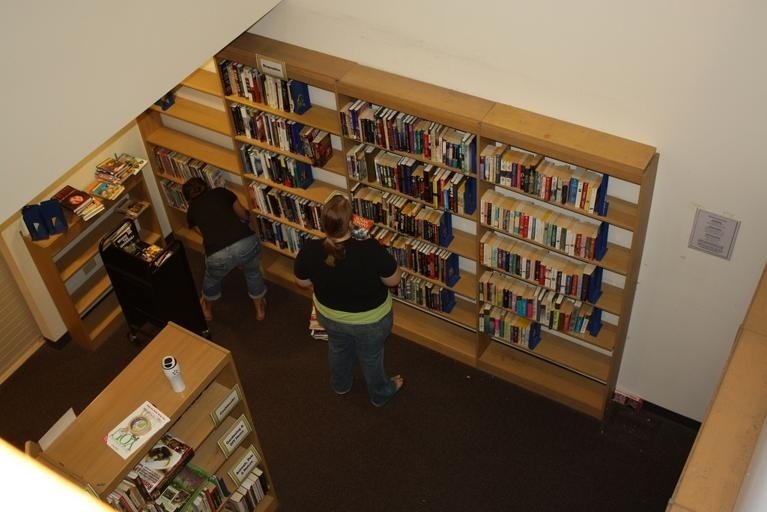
[
  {"left": 294, "top": 195, "right": 404, "bottom": 406},
  {"left": 181, "top": 176, "right": 267, "bottom": 318}
]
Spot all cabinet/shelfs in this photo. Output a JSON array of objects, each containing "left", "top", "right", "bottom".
[
  {"left": 478, "top": 97, "right": 660, "bottom": 419},
  {"left": 337, "top": 64, "right": 495, "bottom": 369},
  {"left": 135, "top": 57, "right": 253, "bottom": 278},
  {"left": 213, "top": 32, "right": 349, "bottom": 329},
  {"left": 19, "top": 158, "right": 164, "bottom": 348},
  {"left": 34, "top": 320, "right": 279, "bottom": 512}
]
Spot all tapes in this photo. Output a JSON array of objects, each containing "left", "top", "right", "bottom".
[{"left": 128, "top": 415, "right": 152, "bottom": 436}]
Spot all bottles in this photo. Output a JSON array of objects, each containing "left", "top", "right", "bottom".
[{"left": 161, "top": 356, "right": 186, "bottom": 392}]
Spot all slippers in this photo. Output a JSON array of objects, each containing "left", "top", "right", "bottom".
[
  {"left": 199, "top": 294, "right": 214, "bottom": 320},
  {"left": 255, "top": 299, "right": 266, "bottom": 321},
  {"left": 371, "top": 375, "right": 409, "bottom": 407},
  {"left": 332, "top": 385, "right": 351, "bottom": 394}
]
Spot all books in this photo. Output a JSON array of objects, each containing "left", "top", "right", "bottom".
[
  {"left": 217, "top": 59, "right": 332, "bottom": 252},
  {"left": 53, "top": 152, "right": 171, "bottom": 265},
  {"left": 106, "top": 381, "right": 268, "bottom": 512},
  {"left": 478, "top": 143, "right": 609, "bottom": 351},
  {"left": 340, "top": 99, "right": 479, "bottom": 312},
  {"left": 152, "top": 145, "right": 227, "bottom": 209}
]
